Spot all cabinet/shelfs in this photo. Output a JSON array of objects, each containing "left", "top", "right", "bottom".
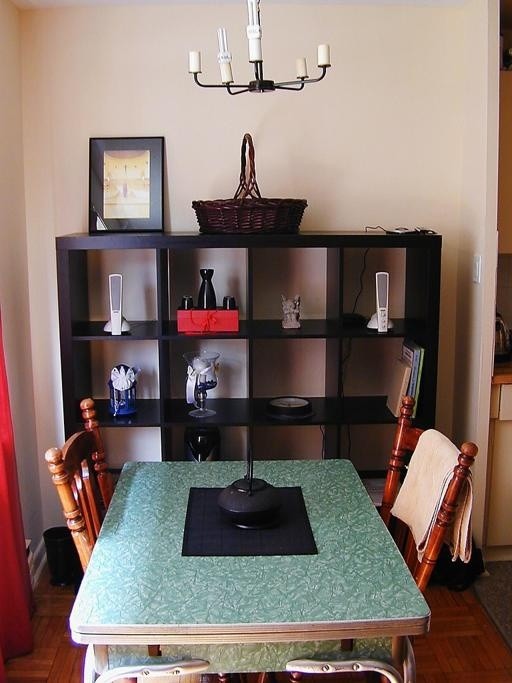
[
  {"left": 56, "top": 232, "right": 441, "bottom": 508},
  {"left": 466, "top": 0, "right": 512, "bottom": 563}
]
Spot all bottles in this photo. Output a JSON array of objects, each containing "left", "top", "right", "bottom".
[{"left": 198, "top": 268, "right": 216, "bottom": 309}]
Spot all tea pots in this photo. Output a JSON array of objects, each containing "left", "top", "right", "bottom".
[{"left": 217, "top": 443, "right": 283, "bottom": 530}]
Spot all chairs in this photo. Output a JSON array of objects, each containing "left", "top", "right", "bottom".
[
  {"left": 382, "top": 397, "right": 478, "bottom": 594},
  {"left": 45, "top": 399, "right": 113, "bottom": 574}
]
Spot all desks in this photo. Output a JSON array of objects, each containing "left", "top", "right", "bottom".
[{"left": 69, "top": 459, "right": 430, "bottom": 683}]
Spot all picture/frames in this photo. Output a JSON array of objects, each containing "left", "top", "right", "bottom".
[{"left": 89, "top": 137, "right": 165, "bottom": 236}]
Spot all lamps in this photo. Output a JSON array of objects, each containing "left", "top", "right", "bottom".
[{"left": 189, "top": 0, "right": 331, "bottom": 95}]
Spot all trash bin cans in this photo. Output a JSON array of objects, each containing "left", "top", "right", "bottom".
[{"left": 43, "top": 527, "right": 82, "bottom": 583}]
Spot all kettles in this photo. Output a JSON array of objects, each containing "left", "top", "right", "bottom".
[{"left": 494, "top": 312, "right": 511, "bottom": 363}]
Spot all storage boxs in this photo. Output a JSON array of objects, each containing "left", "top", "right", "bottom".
[{"left": 176, "top": 306, "right": 240, "bottom": 333}]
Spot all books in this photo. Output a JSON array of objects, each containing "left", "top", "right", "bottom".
[{"left": 386, "top": 338, "right": 426, "bottom": 419}]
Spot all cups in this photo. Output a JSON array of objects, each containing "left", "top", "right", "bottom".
[
  {"left": 223, "top": 295, "right": 236, "bottom": 310},
  {"left": 181, "top": 295, "right": 193, "bottom": 310}
]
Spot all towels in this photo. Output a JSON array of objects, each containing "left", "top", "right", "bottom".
[{"left": 390, "top": 429, "right": 475, "bottom": 564}]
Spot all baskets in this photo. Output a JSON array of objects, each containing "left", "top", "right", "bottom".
[{"left": 189, "top": 130, "right": 310, "bottom": 235}]
